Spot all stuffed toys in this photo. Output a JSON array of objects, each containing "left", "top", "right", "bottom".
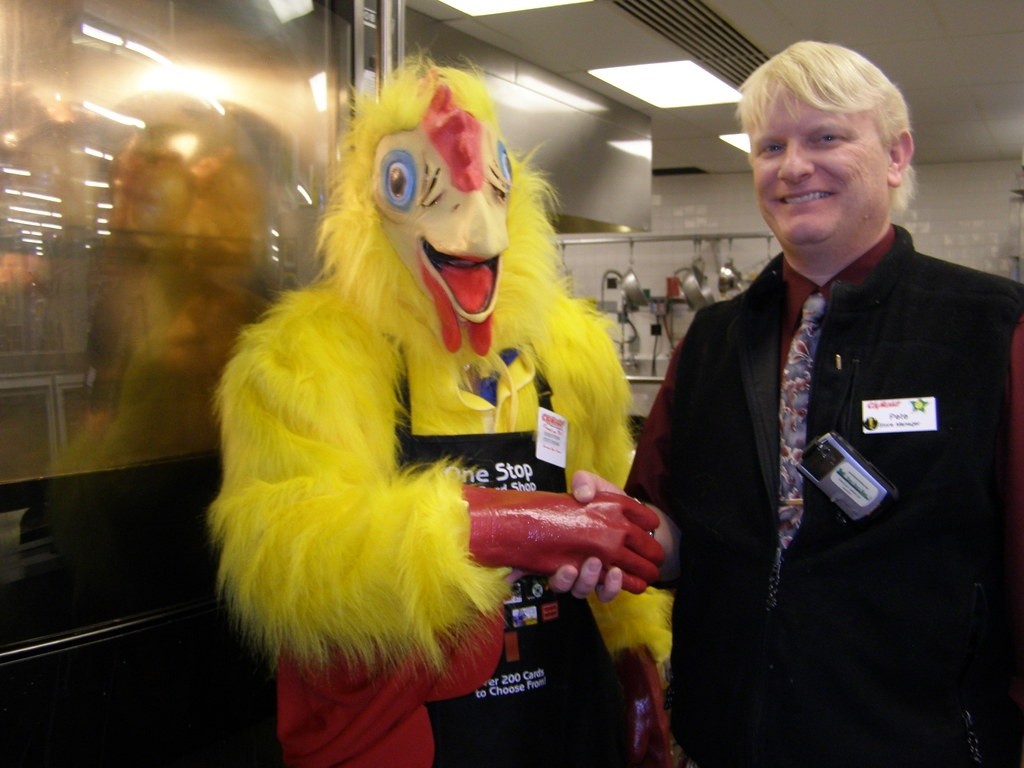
[{"left": 207, "top": 51, "right": 672, "bottom": 767}]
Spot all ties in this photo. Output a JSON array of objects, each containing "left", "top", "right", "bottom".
[{"left": 778, "top": 290, "right": 828, "bottom": 550}]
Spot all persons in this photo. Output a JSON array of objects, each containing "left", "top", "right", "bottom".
[{"left": 547, "top": 40, "right": 1024, "bottom": 767}]
